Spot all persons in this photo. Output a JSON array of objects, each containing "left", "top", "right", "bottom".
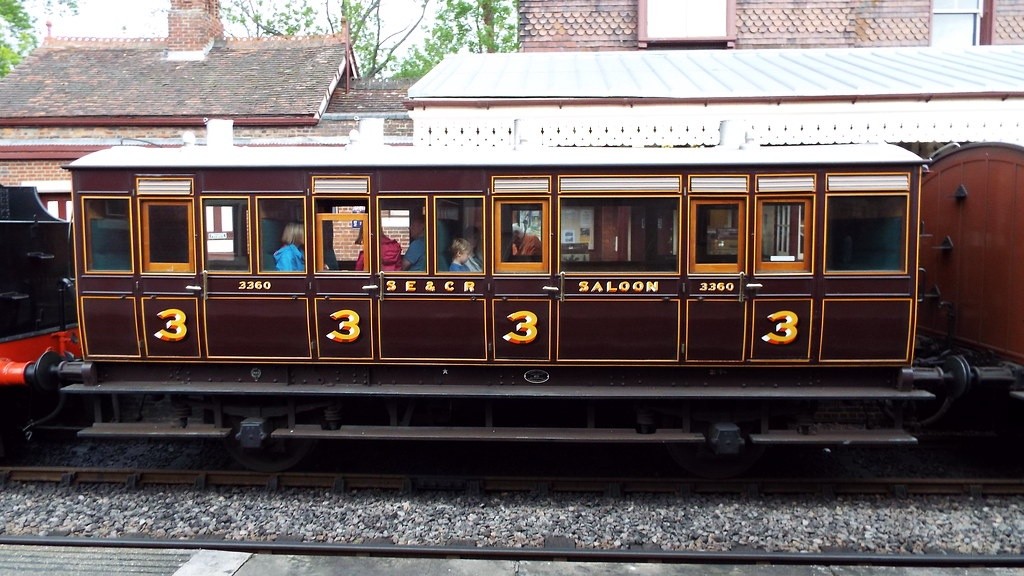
[
  {"left": 402, "top": 213, "right": 427, "bottom": 272},
  {"left": 507, "top": 224, "right": 542, "bottom": 256},
  {"left": 257, "top": 198, "right": 338, "bottom": 273},
  {"left": 459, "top": 227, "right": 484, "bottom": 272},
  {"left": 447, "top": 237, "right": 473, "bottom": 274},
  {"left": 354, "top": 219, "right": 403, "bottom": 272}
]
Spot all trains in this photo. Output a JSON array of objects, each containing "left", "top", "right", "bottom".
[{"left": 0, "top": 133, "right": 1024, "bottom": 471}]
